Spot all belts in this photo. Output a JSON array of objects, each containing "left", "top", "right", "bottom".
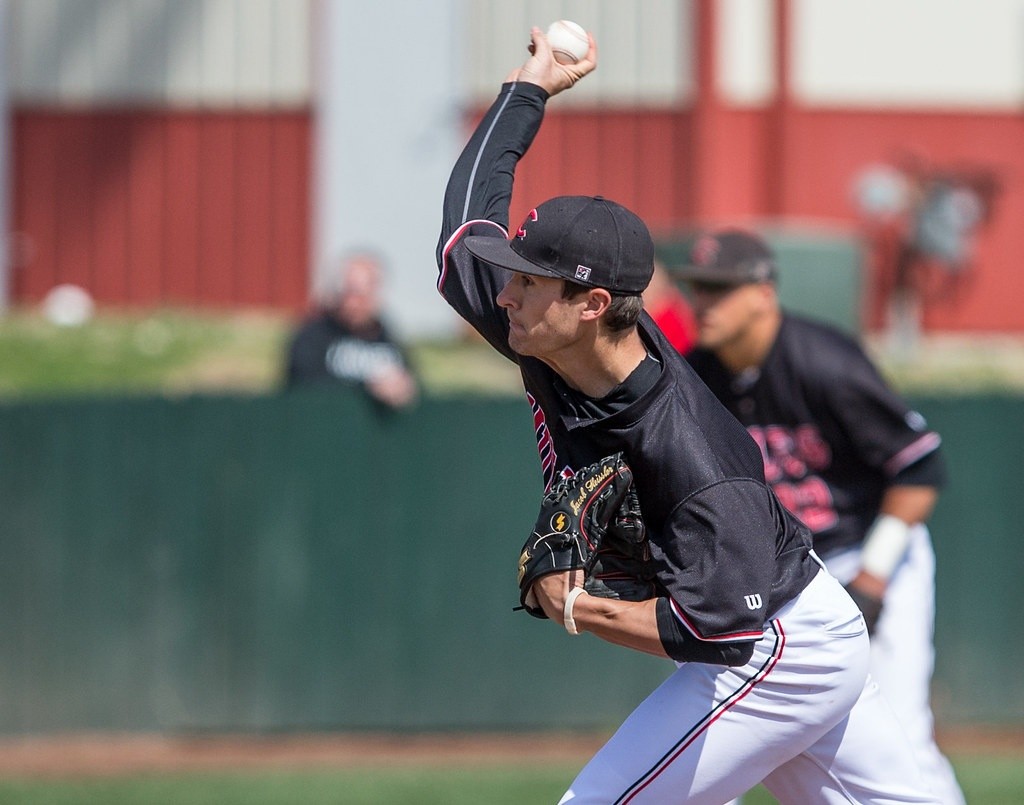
[{"left": 763, "top": 556, "right": 820, "bottom": 623}]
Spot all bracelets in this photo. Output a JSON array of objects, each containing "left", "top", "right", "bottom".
[
  {"left": 859, "top": 514, "right": 912, "bottom": 581},
  {"left": 564, "top": 588, "right": 588, "bottom": 636}
]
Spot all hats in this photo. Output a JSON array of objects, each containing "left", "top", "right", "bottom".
[
  {"left": 465, "top": 194, "right": 655, "bottom": 295},
  {"left": 679, "top": 228, "right": 779, "bottom": 279}
]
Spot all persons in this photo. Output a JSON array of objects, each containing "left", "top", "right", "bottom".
[
  {"left": 436, "top": 24, "right": 960, "bottom": 805},
  {"left": 683, "top": 227, "right": 970, "bottom": 805},
  {"left": 288, "top": 249, "right": 421, "bottom": 411}
]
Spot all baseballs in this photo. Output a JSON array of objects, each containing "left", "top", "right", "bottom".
[{"left": 546, "top": 19, "right": 591, "bottom": 65}]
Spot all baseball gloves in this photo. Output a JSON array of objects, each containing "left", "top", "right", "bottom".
[{"left": 516, "top": 457, "right": 652, "bottom": 619}]
